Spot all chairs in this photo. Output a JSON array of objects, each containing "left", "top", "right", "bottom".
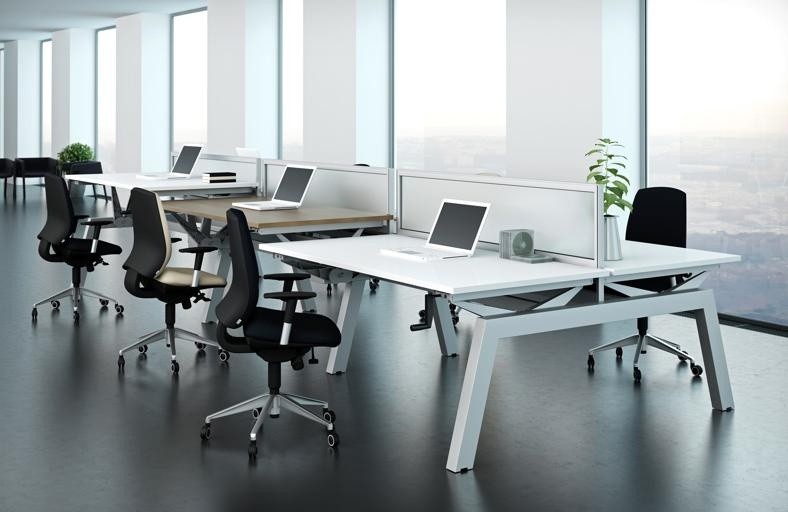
[
  {"left": 30, "top": 173, "right": 124, "bottom": 325},
  {"left": 118, "top": 187, "right": 230, "bottom": 377},
  {"left": 205, "top": 207, "right": 341, "bottom": 456},
  {"left": 588, "top": 187, "right": 704, "bottom": 382},
  {"left": 0, "top": 157, "right": 103, "bottom": 199}
]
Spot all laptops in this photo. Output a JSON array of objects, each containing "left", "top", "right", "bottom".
[
  {"left": 135, "top": 143, "right": 203, "bottom": 181},
  {"left": 379, "top": 198, "right": 491, "bottom": 262},
  {"left": 232, "top": 163, "right": 317, "bottom": 210}
]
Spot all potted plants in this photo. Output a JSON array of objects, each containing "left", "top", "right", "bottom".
[{"left": 585, "top": 137, "right": 635, "bottom": 263}]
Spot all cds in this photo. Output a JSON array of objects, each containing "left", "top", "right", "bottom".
[{"left": 513, "top": 231, "right": 533, "bottom": 256}]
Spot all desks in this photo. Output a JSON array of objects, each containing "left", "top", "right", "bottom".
[
  {"left": 159, "top": 197, "right": 394, "bottom": 324},
  {"left": 65, "top": 176, "right": 259, "bottom": 295},
  {"left": 259, "top": 233, "right": 741, "bottom": 471}
]
